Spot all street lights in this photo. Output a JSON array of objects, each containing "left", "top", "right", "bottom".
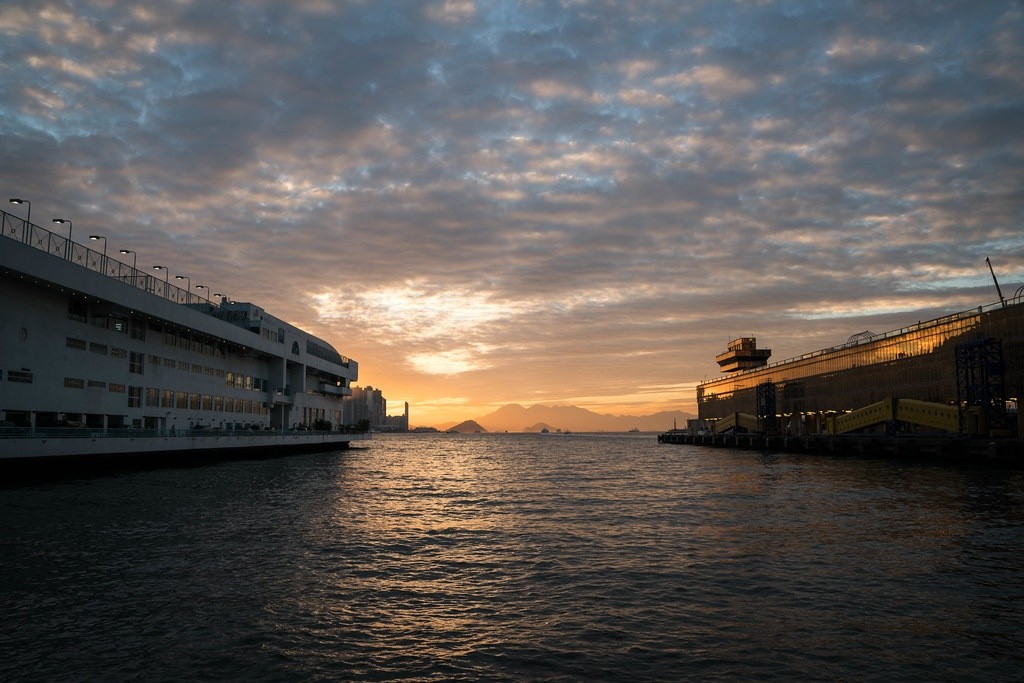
[
  {"left": 153, "top": 266, "right": 169, "bottom": 297},
  {"left": 10, "top": 199, "right": 31, "bottom": 245},
  {"left": 120, "top": 249, "right": 137, "bottom": 286},
  {"left": 176, "top": 276, "right": 191, "bottom": 302},
  {"left": 214, "top": 293, "right": 227, "bottom": 300},
  {"left": 89, "top": 235, "right": 107, "bottom": 275},
  {"left": 52, "top": 219, "right": 72, "bottom": 261},
  {"left": 196, "top": 285, "right": 210, "bottom": 301}
]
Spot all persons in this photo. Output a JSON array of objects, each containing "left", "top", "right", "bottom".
[{"left": 171, "top": 424, "right": 176, "bottom": 436}]
